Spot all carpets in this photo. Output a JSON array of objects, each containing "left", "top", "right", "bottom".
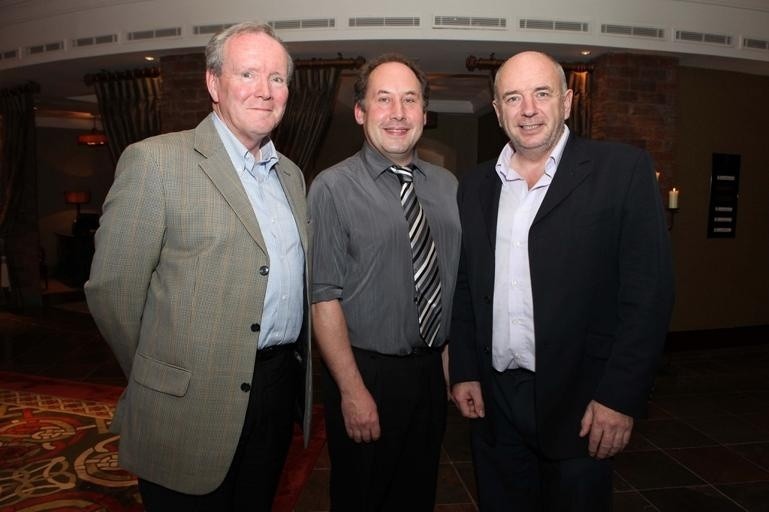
[{"left": 0, "top": 371, "right": 333, "bottom": 510}]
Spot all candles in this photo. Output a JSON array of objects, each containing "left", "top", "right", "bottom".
[{"left": 668, "top": 186, "right": 679, "bottom": 209}]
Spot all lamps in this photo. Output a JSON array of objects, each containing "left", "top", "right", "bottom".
[
  {"left": 64, "top": 190, "right": 91, "bottom": 225},
  {"left": 77, "top": 113, "right": 109, "bottom": 147}
]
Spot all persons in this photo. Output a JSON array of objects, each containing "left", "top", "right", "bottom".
[
  {"left": 448, "top": 49, "right": 678, "bottom": 511},
  {"left": 83, "top": 20, "right": 311, "bottom": 510},
  {"left": 308, "top": 54, "right": 462, "bottom": 511}
]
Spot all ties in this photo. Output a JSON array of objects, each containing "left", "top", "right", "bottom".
[{"left": 389, "top": 164, "right": 444, "bottom": 349}]
